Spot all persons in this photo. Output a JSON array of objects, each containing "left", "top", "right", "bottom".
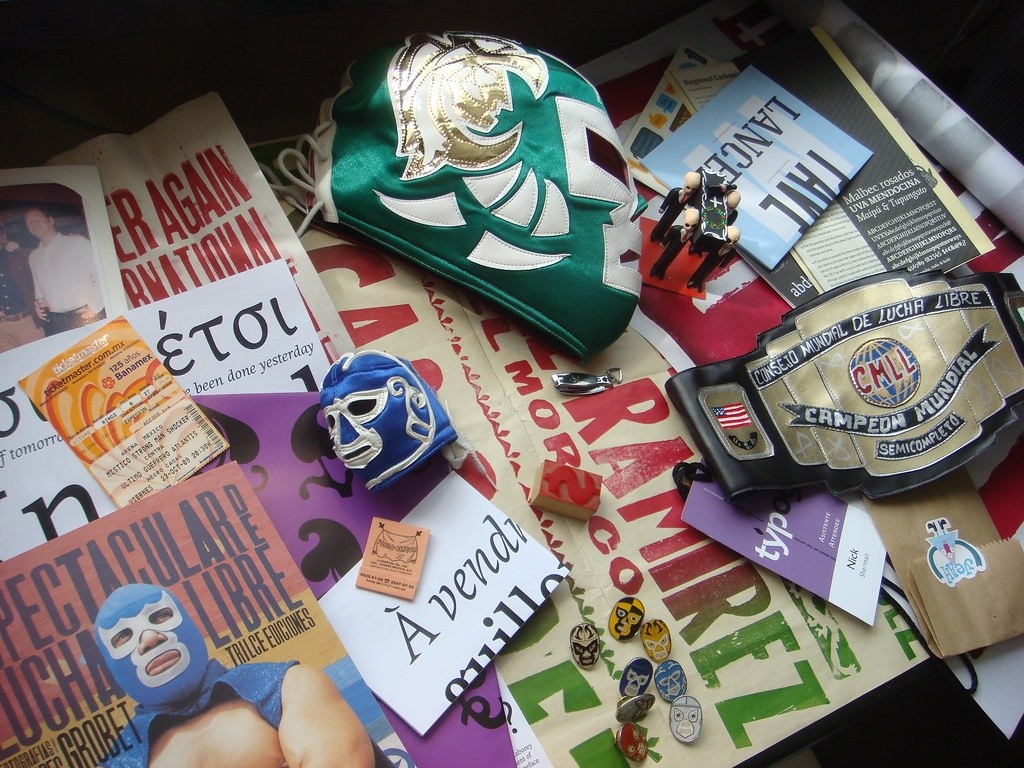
[
  {"left": 92, "top": 583, "right": 378, "bottom": 768},
  {"left": 0, "top": 218, "right": 46, "bottom": 354},
  {"left": 649, "top": 169, "right": 740, "bottom": 294},
  {"left": 19, "top": 207, "right": 107, "bottom": 337}
]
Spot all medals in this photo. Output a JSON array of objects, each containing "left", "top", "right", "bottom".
[{"left": 665, "top": 266, "right": 1023, "bottom": 501}]
